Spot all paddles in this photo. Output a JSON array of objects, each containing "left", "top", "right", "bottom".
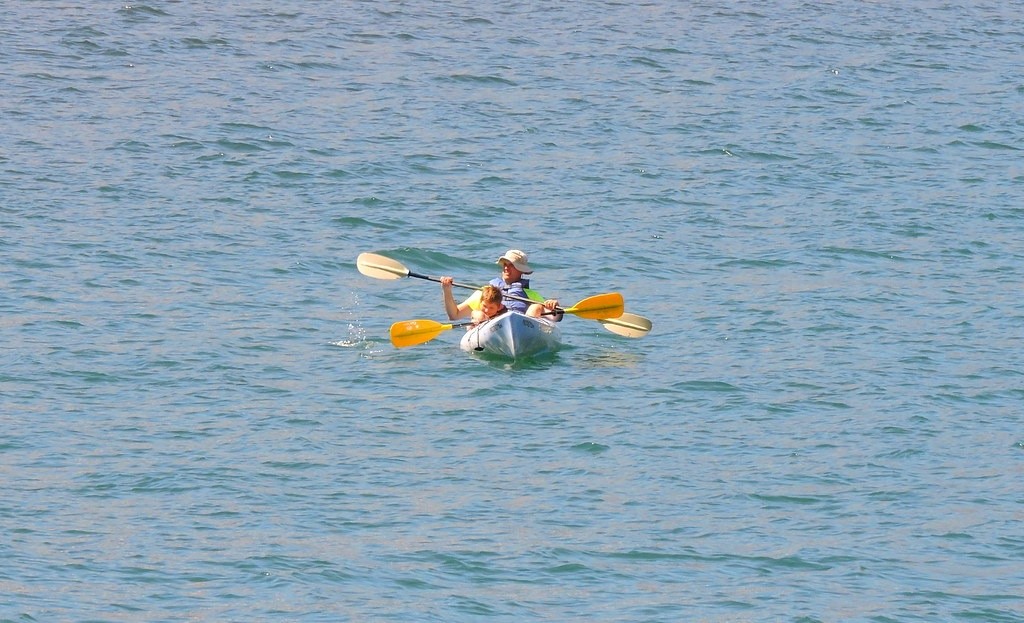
[
  {"left": 390, "top": 293, "right": 625, "bottom": 348},
  {"left": 356, "top": 252, "right": 652, "bottom": 338}
]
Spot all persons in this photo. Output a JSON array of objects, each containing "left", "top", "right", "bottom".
[{"left": 440, "top": 249, "right": 560, "bottom": 333}]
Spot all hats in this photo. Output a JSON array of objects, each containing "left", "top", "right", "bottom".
[{"left": 495, "top": 250, "right": 533, "bottom": 275}]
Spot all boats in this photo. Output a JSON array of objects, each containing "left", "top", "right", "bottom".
[{"left": 459, "top": 311, "right": 562, "bottom": 358}]
